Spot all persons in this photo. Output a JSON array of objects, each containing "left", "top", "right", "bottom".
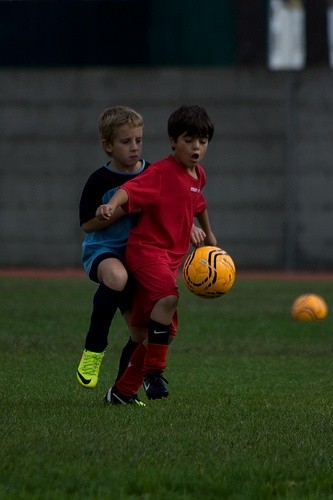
[
  {"left": 77, "top": 107, "right": 207, "bottom": 389},
  {"left": 96, "top": 104, "right": 216, "bottom": 405}
]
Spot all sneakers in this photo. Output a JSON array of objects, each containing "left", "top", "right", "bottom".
[
  {"left": 76, "top": 349, "right": 106, "bottom": 388},
  {"left": 142, "top": 373, "right": 169, "bottom": 400},
  {"left": 103, "top": 384, "right": 146, "bottom": 406}
]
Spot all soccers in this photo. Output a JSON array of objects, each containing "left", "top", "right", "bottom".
[
  {"left": 182, "top": 245, "right": 237, "bottom": 300},
  {"left": 289, "top": 292, "right": 329, "bottom": 322}
]
